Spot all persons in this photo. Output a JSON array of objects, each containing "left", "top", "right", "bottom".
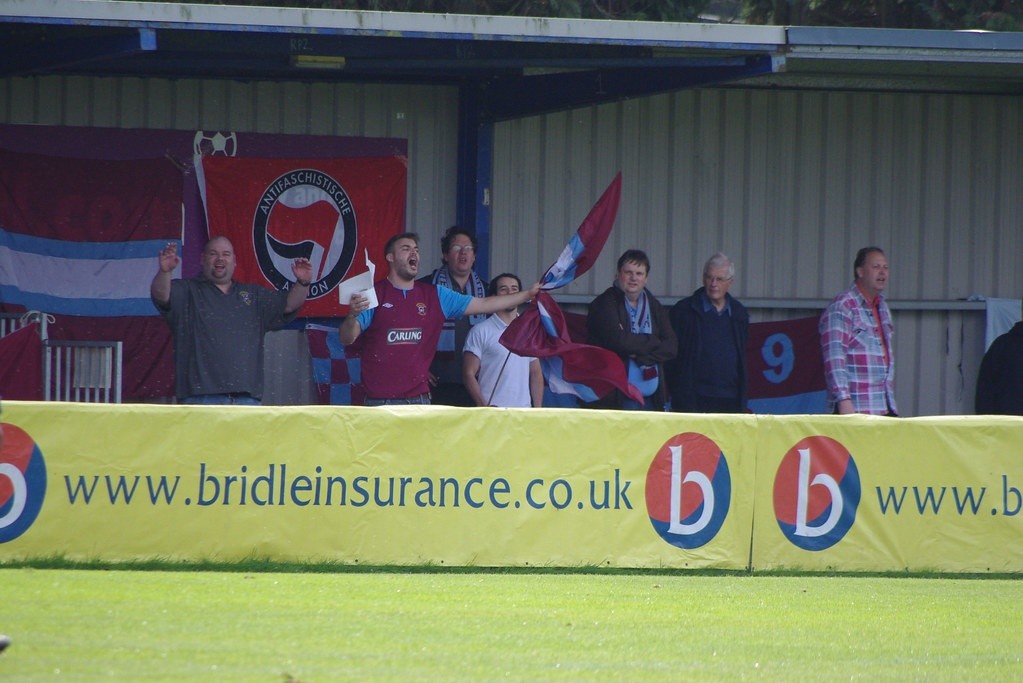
[
  {"left": 585, "top": 249, "right": 678, "bottom": 412},
  {"left": 974, "top": 297, "right": 1023, "bottom": 416},
  {"left": 151, "top": 236, "right": 313, "bottom": 405},
  {"left": 670, "top": 253, "right": 750, "bottom": 413},
  {"left": 339, "top": 228, "right": 544, "bottom": 408},
  {"left": 818, "top": 247, "right": 900, "bottom": 419}
]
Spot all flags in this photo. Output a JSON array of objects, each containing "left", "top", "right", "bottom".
[{"left": 499, "top": 172, "right": 645, "bottom": 407}]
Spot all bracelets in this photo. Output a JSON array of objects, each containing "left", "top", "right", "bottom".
[{"left": 297, "top": 278, "right": 311, "bottom": 287}]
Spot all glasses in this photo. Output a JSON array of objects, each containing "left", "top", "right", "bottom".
[
  {"left": 704, "top": 274, "right": 733, "bottom": 283},
  {"left": 448, "top": 245, "right": 474, "bottom": 253}
]
